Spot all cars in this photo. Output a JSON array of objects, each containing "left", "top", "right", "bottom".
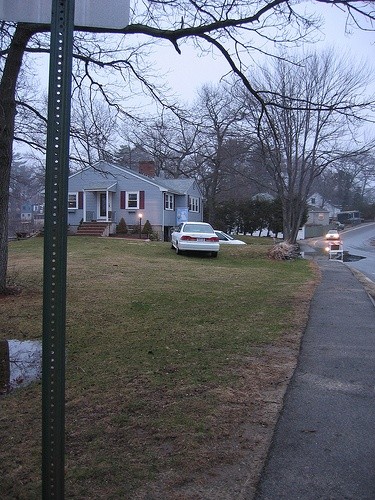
[
  {"left": 170, "top": 221, "right": 220, "bottom": 257},
  {"left": 214, "top": 230, "right": 247, "bottom": 244},
  {"left": 325, "top": 230, "right": 340, "bottom": 240}
]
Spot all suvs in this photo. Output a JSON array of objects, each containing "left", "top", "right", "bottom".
[{"left": 335, "top": 221, "right": 345, "bottom": 231}]
